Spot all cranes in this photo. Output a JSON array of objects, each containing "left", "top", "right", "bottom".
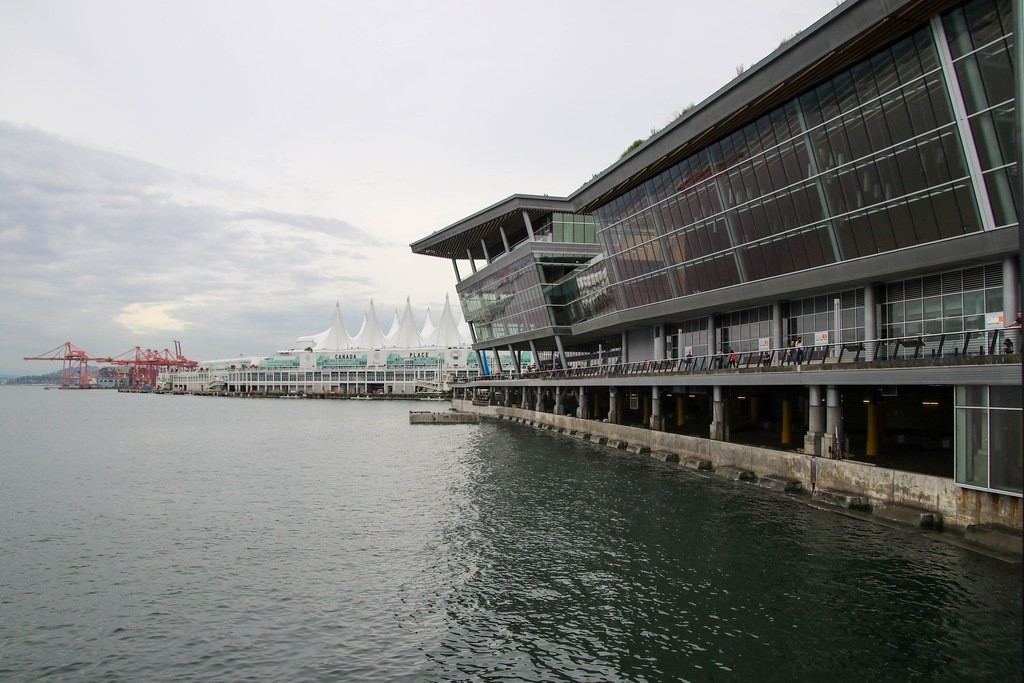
[{"left": 22, "top": 339, "right": 200, "bottom": 392}]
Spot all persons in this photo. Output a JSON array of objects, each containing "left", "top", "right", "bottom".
[
  {"left": 763, "top": 352, "right": 770, "bottom": 367},
  {"left": 686, "top": 351, "right": 693, "bottom": 370},
  {"left": 787, "top": 336, "right": 804, "bottom": 366},
  {"left": 728, "top": 350, "right": 736, "bottom": 368},
  {"left": 644, "top": 360, "right": 649, "bottom": 370},
  {"left": 716, "top": 349, "right": 723, "bottom": 369},
  {"left": 1005, "top": 311, "right": 1021, "bottom": 328},
  {"left": 1004, "top": 338, "right": 1013, "bottom": 354}
]
